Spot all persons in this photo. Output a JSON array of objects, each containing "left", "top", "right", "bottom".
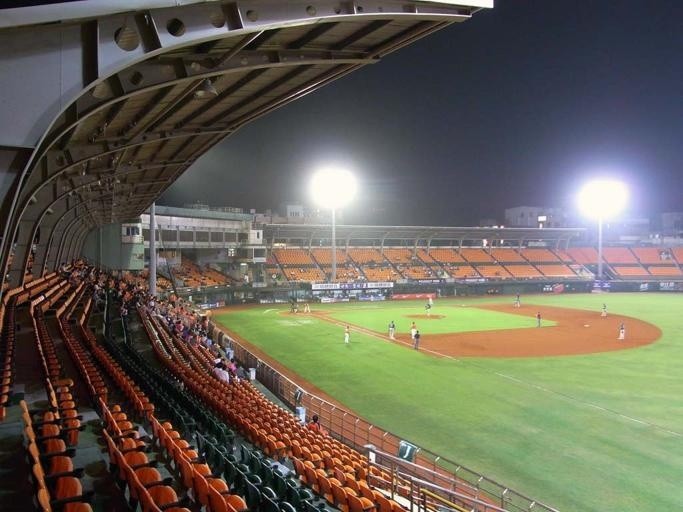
[
  {"left": 344, "top": 325, "right": 351, "bottom": 344},
  {"left": 287, "top": 250, "right": 500, "bottom": 314},
  {"left": 616, "top": 320, "right": 625, "bottom": 340},
  {"left": 412, "top": 331, "right": 420, "bottom": 349},
  {"left": 307, "top": 413, "right": 328, "bottom": 435},
  {"left": 424, "top": 303, "right": 431, "bottom": 315},
  {"left": 57, "top": 254, "right": 246, "bottom": 384},
  {"left": 598, "top": 302, "right": 606, "bottom": 317},
  {"left": 511, "top": 292, "right": 521, "bottom": 308},
  {"left": 535, "top": 311, "right": 541, "bottom": 326},
  {"left": 388, "top": 321, "right": 396, "bottom": 339},
  {"left": 410, "top": 322, "right": 416, "bottom": 338}
]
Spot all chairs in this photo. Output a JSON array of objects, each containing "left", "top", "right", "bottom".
[{"left": 2, "top": 274, "right": 419, "bottom": 510}]
